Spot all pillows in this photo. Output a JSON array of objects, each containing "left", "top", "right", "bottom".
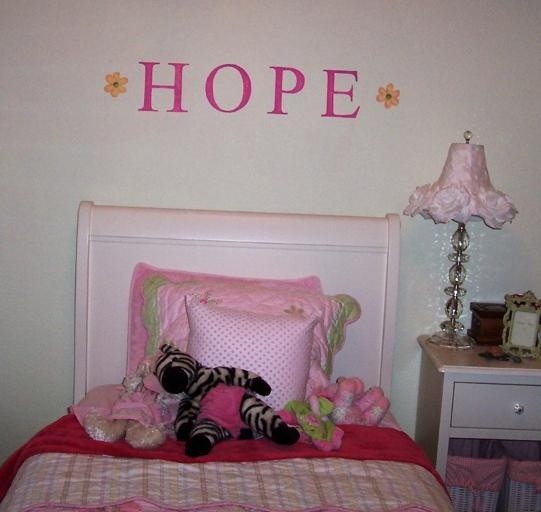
[
  {"left": 126, "top": 262, "right": 321, "bottom": 381},
  {"left": 184, "top": 292, "right": 322, "bottom": 413},
  {"left": 142, "top": 274, "right": 362, "bottom": 414}
]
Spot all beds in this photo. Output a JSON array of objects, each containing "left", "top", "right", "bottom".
[{"left": 0, "top": 200, "right": 454, "bottom": 512}]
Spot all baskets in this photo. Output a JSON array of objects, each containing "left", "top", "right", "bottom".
[
  {"left": 502, "top": 441, "right": 540, "bottom": 512},
  {"left": 442, "top": 440, "right": 507, "bottom": 511}
]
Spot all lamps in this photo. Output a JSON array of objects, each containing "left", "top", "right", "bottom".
[{"left": 403, "top": 130, "right": 520, "bottom": 350}]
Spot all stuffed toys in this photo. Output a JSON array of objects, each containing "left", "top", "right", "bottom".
[{"left": 154, "top": 345, "right": 300, "bottom": 457}]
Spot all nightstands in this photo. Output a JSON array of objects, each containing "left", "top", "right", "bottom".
[{"left": 416, "top": 334, "right": 541, "bottom": 485}]
